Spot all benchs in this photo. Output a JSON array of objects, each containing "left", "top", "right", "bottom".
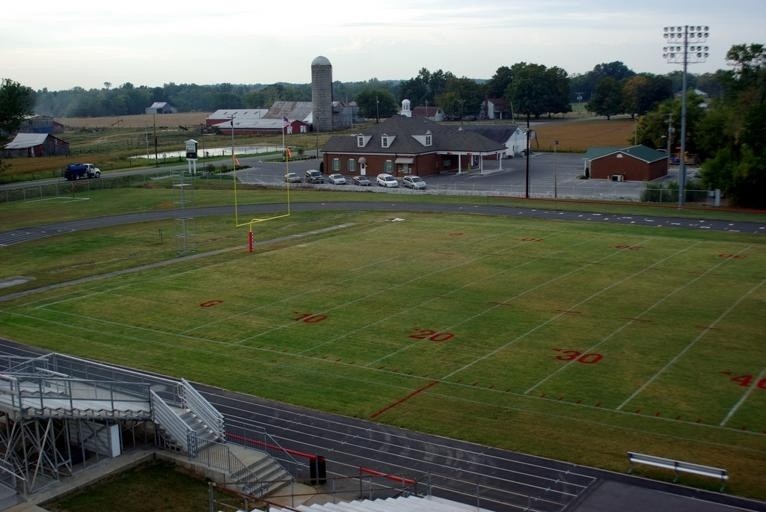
[{"left": 627, "top": 450, "right": 729, "bottom": 493}]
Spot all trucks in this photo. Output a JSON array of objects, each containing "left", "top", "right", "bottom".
[{"left": 62, "top": 162, "right": 101, "bottom": 181}]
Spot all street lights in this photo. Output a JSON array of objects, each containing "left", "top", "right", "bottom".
[{"left": 662, "top": 25, "right": 711, "bottom": 211}]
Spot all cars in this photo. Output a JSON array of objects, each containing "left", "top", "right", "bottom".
[{"left": 284, "top": 169, "right": 427, "bottom": 190}]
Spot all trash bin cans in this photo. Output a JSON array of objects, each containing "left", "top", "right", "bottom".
[{"left": 310, "top": 455, "right": 327, "bottom": 485}]
